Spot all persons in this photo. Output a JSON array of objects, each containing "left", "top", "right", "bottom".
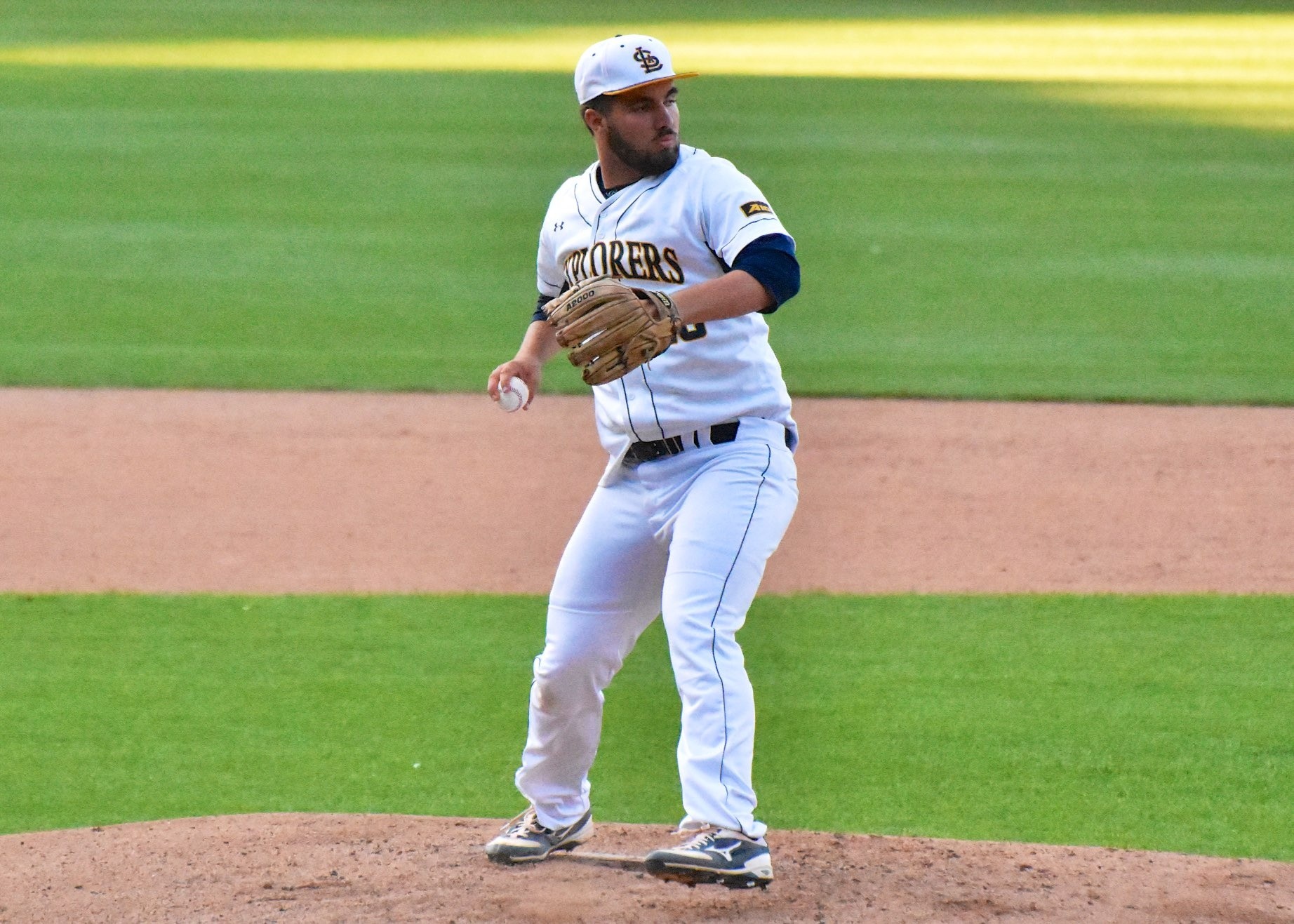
[{"left": 486, "top": 34, "right": 799, "bottom": 890}]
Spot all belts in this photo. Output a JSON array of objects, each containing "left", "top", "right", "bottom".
[{"left": 621, "top": 420, "right": 791, "bottom": 461}]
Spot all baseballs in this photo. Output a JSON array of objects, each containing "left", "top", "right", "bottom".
[{"left": 494, "top": 376, "right": 529, "bottom": 413}]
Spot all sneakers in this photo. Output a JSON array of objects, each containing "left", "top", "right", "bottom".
[
  {"left": 485, "top": 806, "right": 593, "bottom": 868},
  {"left": 642, "top": 824, "right": 775, "bottom": 891}
]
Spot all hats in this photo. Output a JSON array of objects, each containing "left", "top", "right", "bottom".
[{"left": 574, "top": 33, "right": 700, "bottom": 107}]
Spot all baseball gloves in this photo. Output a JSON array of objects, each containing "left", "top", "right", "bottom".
[{"left": 541, "top": 274, "right": 683, "bottom": 386}]
